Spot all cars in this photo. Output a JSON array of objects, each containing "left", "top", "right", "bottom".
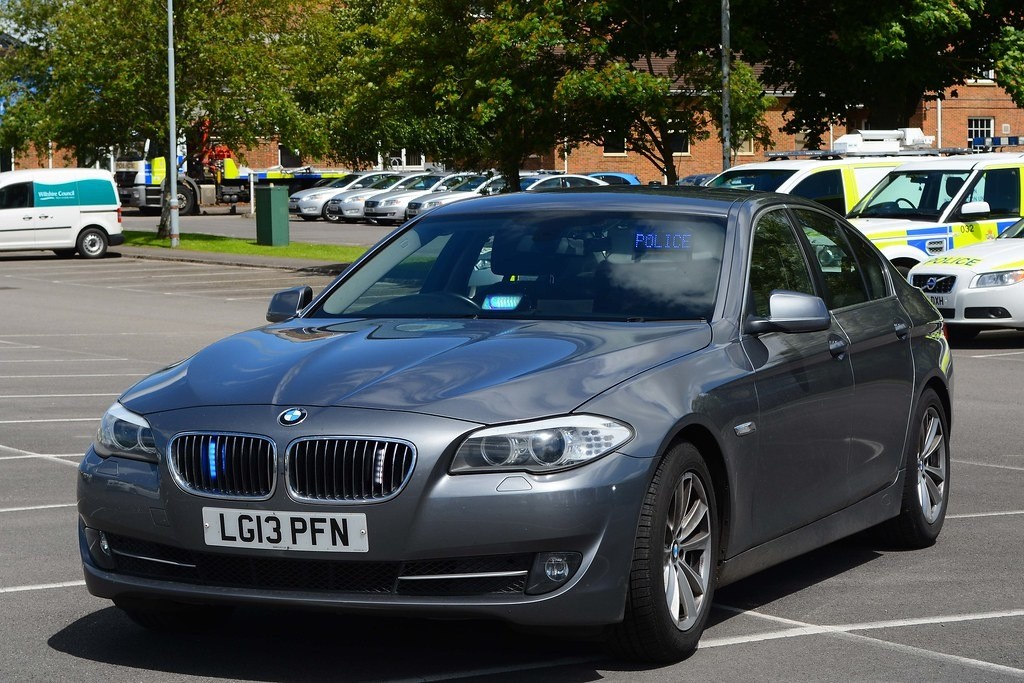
[
  {"left": 288, "top": 169, "right": 641, "bottom": 225},
  {"left": 77, "top": 188, "right": 957, "bottom": 657},
  {"left": 675, "top": 173, "right": 719, "bottom": 187},
  {"left": 905, "top": 217, "right": 1024, "bottom": 337}
]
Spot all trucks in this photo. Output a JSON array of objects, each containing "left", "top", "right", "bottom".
[{"left": 114, "top": 129, "right": 353, "bottom": 217}]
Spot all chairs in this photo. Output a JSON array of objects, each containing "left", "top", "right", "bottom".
[
  {"left": 19, "top": 191, "right": 33, "bottom": 208},
  {"left": 938, "top": 177, "right": 970, "bottom": 218},
  {"left": 474, "top": 228, "right": 564, "bottom": 314}
]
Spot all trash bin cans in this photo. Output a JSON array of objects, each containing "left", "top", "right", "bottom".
[{"left": 254, "top": 186, "right": 290, "bottom": 246}]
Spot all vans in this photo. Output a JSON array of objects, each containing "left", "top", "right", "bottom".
[
  {"left": 703, "top": 127, "right": 1024, "bottom": 280},
  {"left": 0, "top": 168, "right": 125, "bottom": 259}
]
